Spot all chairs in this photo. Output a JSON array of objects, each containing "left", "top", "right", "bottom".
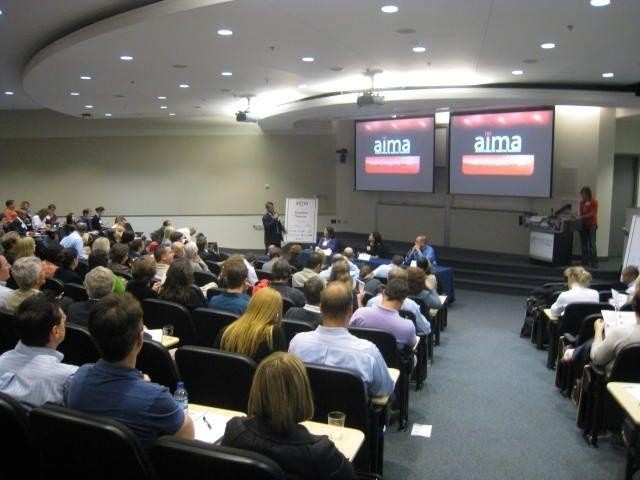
[
  {"left": 519, "top": 280, "right": 640, "bottom": 447},
  {"left": 0, "top": 216, "right": 455, "bottom": 480}
]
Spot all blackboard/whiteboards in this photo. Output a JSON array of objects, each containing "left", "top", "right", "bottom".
[
  {"left": 620, "top": 214, "right": 640, "bottom": 283},
  {"left": 284, "top": 198, "right": 319, "bottom": 245}
]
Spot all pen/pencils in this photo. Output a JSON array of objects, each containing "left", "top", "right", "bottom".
[{"left": 203, "top": 416, "right": 212, "bottom": 429}]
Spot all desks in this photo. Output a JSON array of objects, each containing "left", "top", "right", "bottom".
[{"left": 606, "top": 382, "right": 640, "bottom": 479}]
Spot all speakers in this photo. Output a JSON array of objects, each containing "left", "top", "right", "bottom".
[{"left": 339, "top": 152, "right": 349, "bottom": 166}]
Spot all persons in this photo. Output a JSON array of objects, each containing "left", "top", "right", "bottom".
[
  {"left": 576, "top": 187, "right": 597, "bottom": 268},
  {"left": 551, "top": 266, "right": 640, "bottom": 379},
  {"left": 0, "top": 199, "right": 435, "bottom": 479}
]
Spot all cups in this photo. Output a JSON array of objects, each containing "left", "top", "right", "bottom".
[
  {"left": 328, "top": 410, "right": 345, "bottom": 442},
  {"left": 163, "top": 324, "right": 174, "bottom": 336}
]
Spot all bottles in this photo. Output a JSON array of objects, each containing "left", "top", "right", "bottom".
[
  {"left": 174, "top": 382, "right": 189, "bottom": 414},
  {"left": 432, "top": 261, "right": 437, "bottom": 273}
]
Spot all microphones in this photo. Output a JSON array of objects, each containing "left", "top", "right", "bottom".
[{"left": 403, "top": 244, "right": 414, "bottom": 265}]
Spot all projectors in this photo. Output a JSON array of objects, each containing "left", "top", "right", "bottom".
[
  {"left": 357, "top": 95, "right": 385, "bottom": 107},
  {"left": 236, "top": 113, "right": 258, "bottom": 123}
]
